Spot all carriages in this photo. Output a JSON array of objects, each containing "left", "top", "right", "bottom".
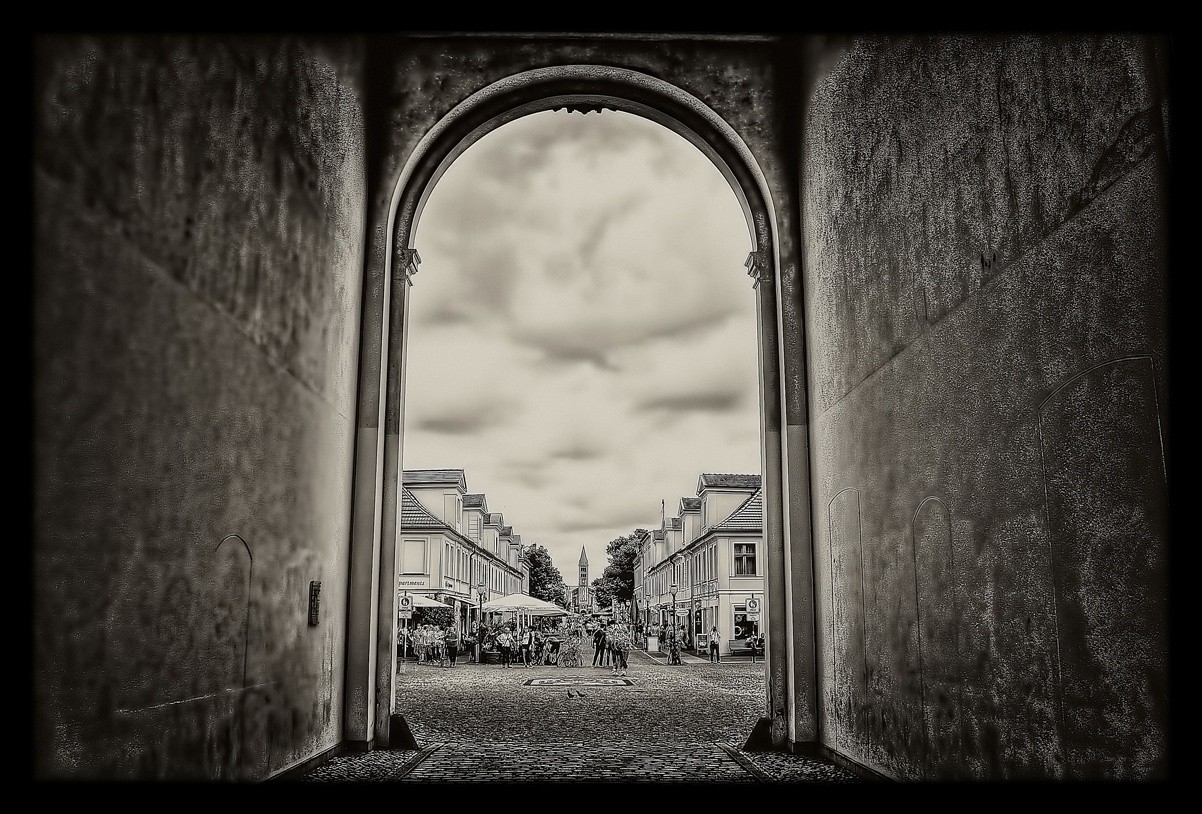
[{"left": 602, "top": 618, "right": 633, "bottom": 676}]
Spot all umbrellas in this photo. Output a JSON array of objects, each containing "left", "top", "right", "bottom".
[
  {"left": 471, "top": 593, "right": 572, "bottom": 650},
  {"left": 399, "top": 592, "right": 453, "bottom": 608}
]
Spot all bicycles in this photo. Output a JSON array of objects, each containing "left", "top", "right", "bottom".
[
  {"left": 438, "top": 638, "right": 448, "bottom": 667},
  {"left": 530, "top": 640, "right": 583, "bottom": 669}
]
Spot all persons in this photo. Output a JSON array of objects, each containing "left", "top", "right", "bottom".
[
  {"left": 497, "top": 627, "right": 516, "bottom": 669},
  {"left": 746, "top": 633, "right": 764, "bottom": 648},
  {"left": 710, "top": 628, "right": 721, "bottom": 663},
  {"left": 547, "top": 637, "right": 560, "bottom": 654},
  {"left": 521, "top": 625, "right": 536, "bottom": 669},
  {"left": 413, "top": 623, "right": 458, "bottom": 668},
  {"left": 592, "top": 624, "right": 630, "bottom": 671},
  {"left": 638, "top": 621, "right": 668, "bottom": 649},
  {"left": 551, "top": 619, "right": 556, "bottom": 632}
]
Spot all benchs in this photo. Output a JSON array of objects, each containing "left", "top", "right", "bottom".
[{"left": 729, "top": 639, "right": 763, "bottom": 656}]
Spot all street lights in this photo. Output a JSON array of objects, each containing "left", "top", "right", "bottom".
[
  {"left": 626, "top": 599, "right": 631, "bottom": 625},
  {"left": 646, "top": 594, "right": 650, "bottom": 637},
  {"left": 667, "top": 583, "right": 679, "bottom": 645},
  {"left": 476, "top": 582, "right": 485, "bottom": 664}
]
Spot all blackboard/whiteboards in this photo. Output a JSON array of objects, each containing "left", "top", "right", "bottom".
[{"left": 696, "top": 634, "right": 710, "bottom": 650}]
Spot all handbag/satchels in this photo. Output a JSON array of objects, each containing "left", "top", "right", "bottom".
[{"left": 711, "top": 640, "right": 715, "bottom": 645}]
